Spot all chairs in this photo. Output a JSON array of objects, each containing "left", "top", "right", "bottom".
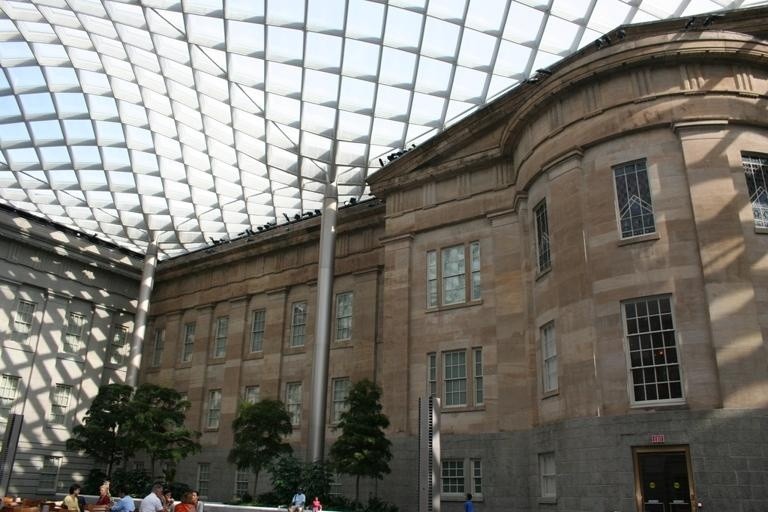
[{"left": 0, "top": 496, "right": 139, "bottom": 512}]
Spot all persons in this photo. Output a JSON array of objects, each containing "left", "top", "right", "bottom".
[
  {"left": 288, "top": 489, "right": 306, "bottom": 512},
  {"left": 61, "top": 481, "right": 204, "bottom": 512},
  {"left": 464, "top": 493, "right": 474, "bottom": 512},
  {"left": 312, "top": 496, "right": 320, "bottom": 512}
]
